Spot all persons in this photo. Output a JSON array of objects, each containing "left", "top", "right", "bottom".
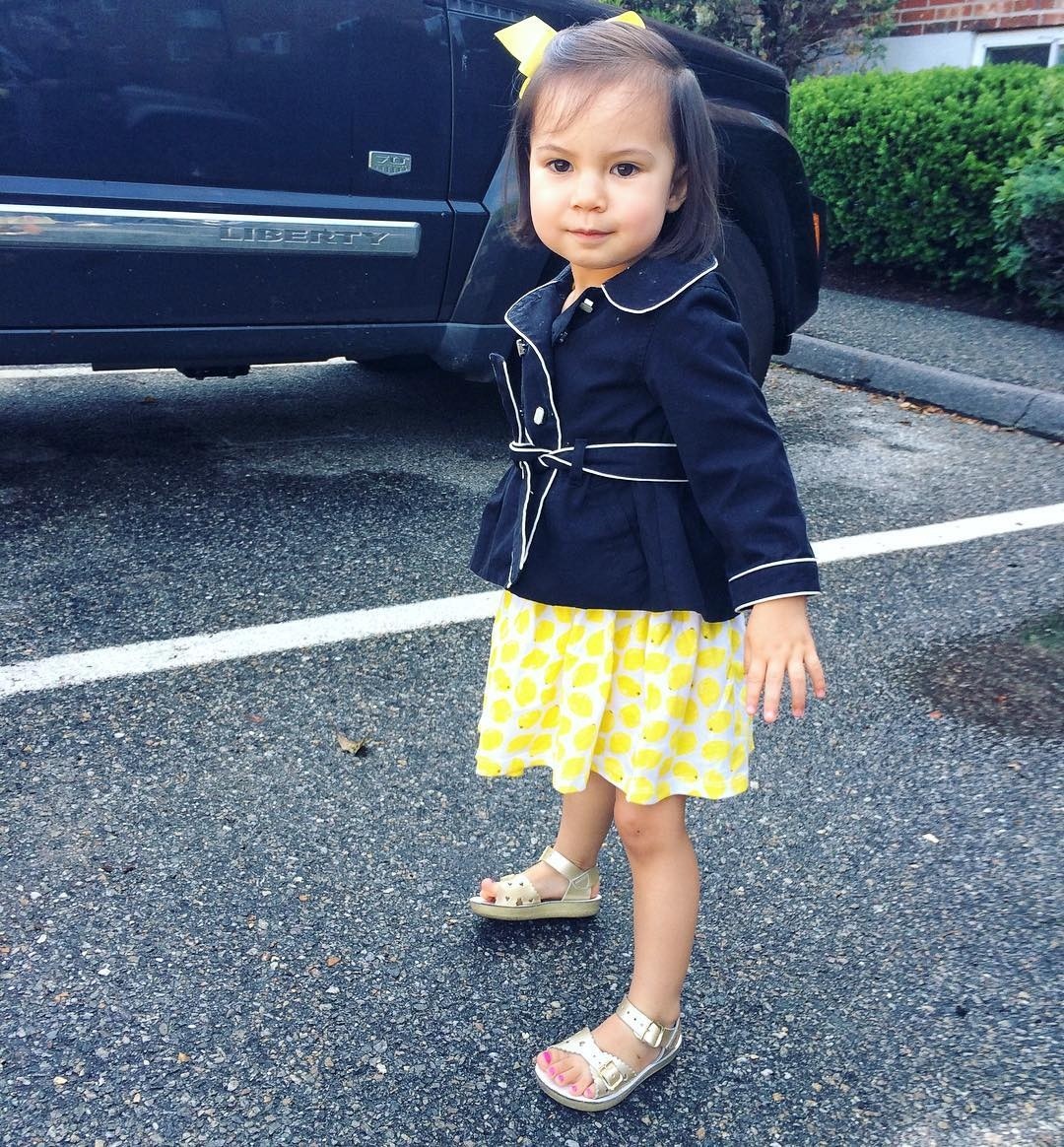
[{"left": 467, "top": 12, "right": 826, "bottom": 1112}]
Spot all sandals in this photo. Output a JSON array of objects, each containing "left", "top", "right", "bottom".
[
  {"left": 534, "top": 995, "right": 682, "bottom": 1111},
  {"left": 470, "top": 846, "right": 601, "bottom": 921}
]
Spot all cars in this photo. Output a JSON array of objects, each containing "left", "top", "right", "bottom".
[{"left": 0, "top": 0, "right": 833, "bottom": 440}]
